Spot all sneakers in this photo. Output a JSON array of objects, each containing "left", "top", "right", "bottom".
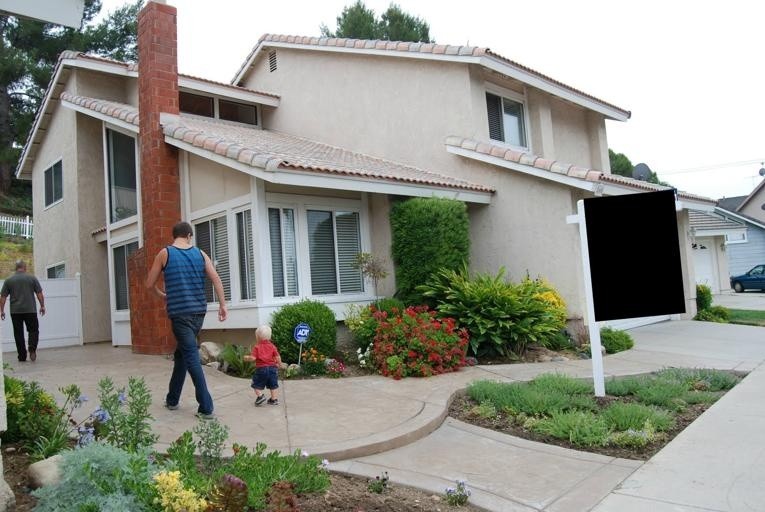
[
  {"left": 255, "top": 393, "right": 266, "bottom": 404},
  {"left": 28, "top": 346, "right": 37, "bottom": 361},
  {"left": 267, "top": 398, "right": 279, "bottom": 405},
  {"left": 165, "top": 403, "right": 216, "bottom": 419}
]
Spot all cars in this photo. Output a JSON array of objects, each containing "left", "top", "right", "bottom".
[{"left": 729, "top": 264, "right": 765, "bottom": 293}]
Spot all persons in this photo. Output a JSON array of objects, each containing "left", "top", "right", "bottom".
[
  {"left": 758, "top": 266, "right": 765, "bottom": 275},
  {"left": 244, "top": 325, "right": 286, "bottom": 405},
  {"left": 0, "top": 261, "right": 46, "bottom": 360},
  {"left": 145, "top": 223, "right": 226, "bottom": 414}
]
